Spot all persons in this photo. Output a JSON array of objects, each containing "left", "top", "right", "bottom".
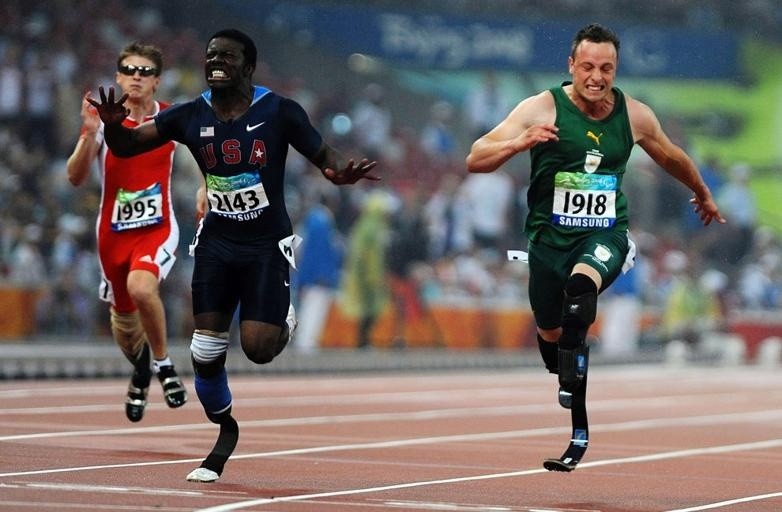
[
  {"left": 465, "top": 21, "right": 727, "bottom": 473},
  {"left": 1, "top": 1, "right": 781, "bottom": 357},
  {"left": 84, "top": 29, "right": 383, "bottom": 482},
  {"left": 66, "top": 39, "right": 210, "bottom": 423}
]
